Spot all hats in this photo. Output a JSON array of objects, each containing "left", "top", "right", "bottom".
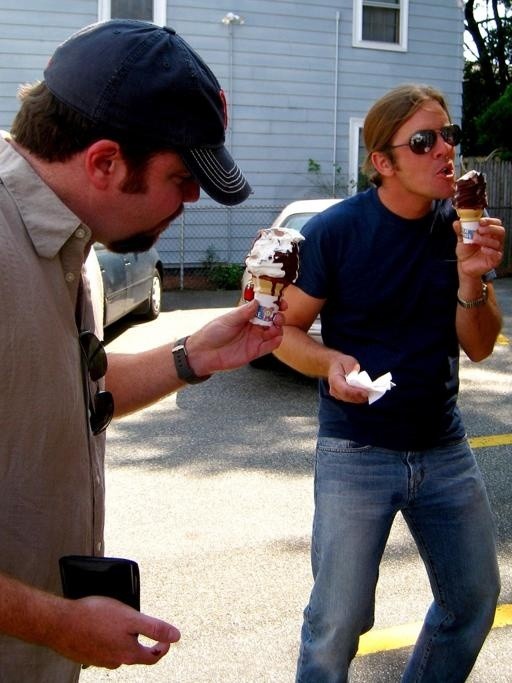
[{"left": 45, "top": 20, "right": 251, "bottom": 205}]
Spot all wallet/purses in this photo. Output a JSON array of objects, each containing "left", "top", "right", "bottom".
[{"left": 59, "top": 556, "right": 140, "bottom": 612}]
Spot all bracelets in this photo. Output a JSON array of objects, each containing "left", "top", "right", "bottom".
[{"left": 171, "top": 334, "right": 213, "bottom": 384}]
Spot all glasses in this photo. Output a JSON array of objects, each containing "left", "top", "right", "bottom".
[
  {"left": 79, "top": 329, "right": 113, "bottom": 435},
  {"left": 392, "top": 124, "right": 461, "bottom": 153}
]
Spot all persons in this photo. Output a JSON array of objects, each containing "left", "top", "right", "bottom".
[
  {"left": 267, "top": 81, "right": 506, "bottom": 683},
  {"left": 0, "top": 17, "right": 289, "bottom": 683}
]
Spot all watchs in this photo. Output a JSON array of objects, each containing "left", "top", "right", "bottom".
[{"left": 456, "top": 281, "right": 488, "bottom": 309}]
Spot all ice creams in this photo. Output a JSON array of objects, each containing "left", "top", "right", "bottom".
[
  {"left": 245, "top": 227, "right": 307, "bottom": 326},
  {"left": 449, "top": 170, "right": 488, "bottom": 245}
]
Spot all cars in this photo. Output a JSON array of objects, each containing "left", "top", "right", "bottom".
[
  {"left": 240, "top": 198, "right": 354, "bottom": 371},
  {"left": 75, "top": 237, "right": 164, "bottom": 328}
]
